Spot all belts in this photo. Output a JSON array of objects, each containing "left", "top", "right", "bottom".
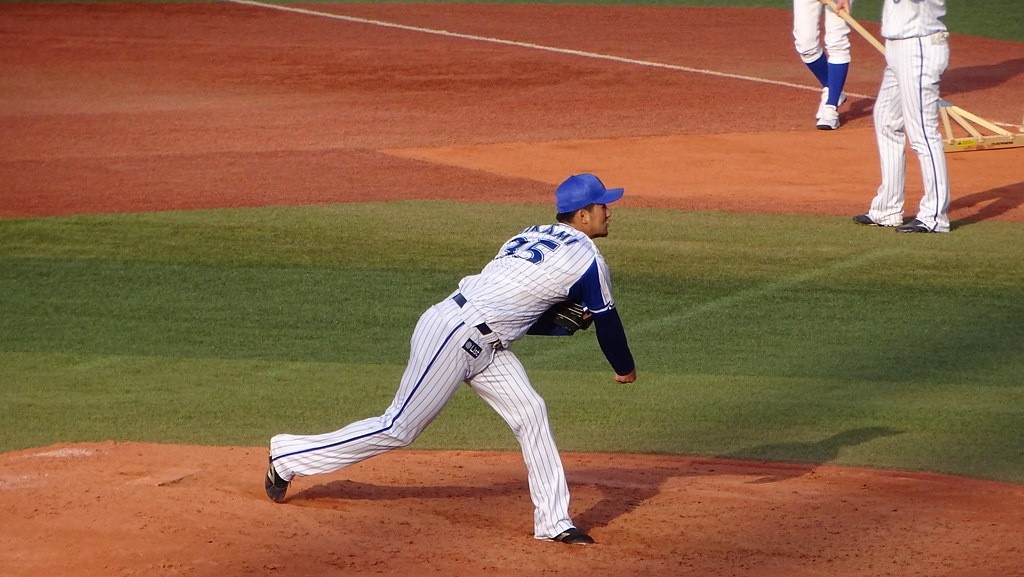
[{"left": 453, "top": 293, "right": 503, "bottom": 350}]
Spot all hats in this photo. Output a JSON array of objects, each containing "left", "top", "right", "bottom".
[{"left": 554, "top": 173, "right": 624, "bottom": 213}]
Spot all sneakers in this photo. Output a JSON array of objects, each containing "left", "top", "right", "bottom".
[
  {"left": 816, "top": 104, "right": 840, "bottom": 130},
  {"left": 852, "top": 212, "right": 885, "bottom": 227},
  {"left": 265, "top": 442, "right": 289, "bottom": 503},
  {"left": 546, "top": 527, "right": 595, "bottom": 545},
  {"left": 895, "top": 218, "right": 938, "bottom": 233},
  {"left": 816, "top": 87, "right": 847, "bottom": 119}
]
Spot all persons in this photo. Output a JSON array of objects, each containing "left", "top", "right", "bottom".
[
  {"left": 853, "top": 0, "right": 950, "bottom": 233},
  {"left": 789, "top": 0, "right": 853, "bottom": 130},
  {"left": 265, "top": 173, "right": 637, "bottom": 546}
]
